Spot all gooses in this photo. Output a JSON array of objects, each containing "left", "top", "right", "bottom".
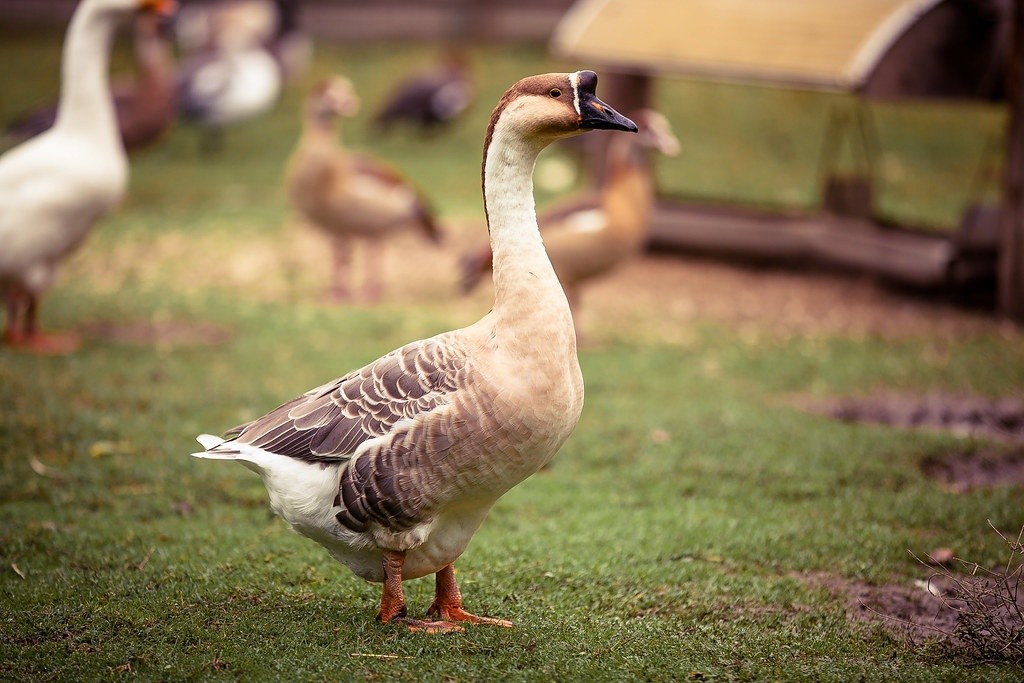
[{"left": 1, "top": 0, "right": 684, "bottom": 634}]
